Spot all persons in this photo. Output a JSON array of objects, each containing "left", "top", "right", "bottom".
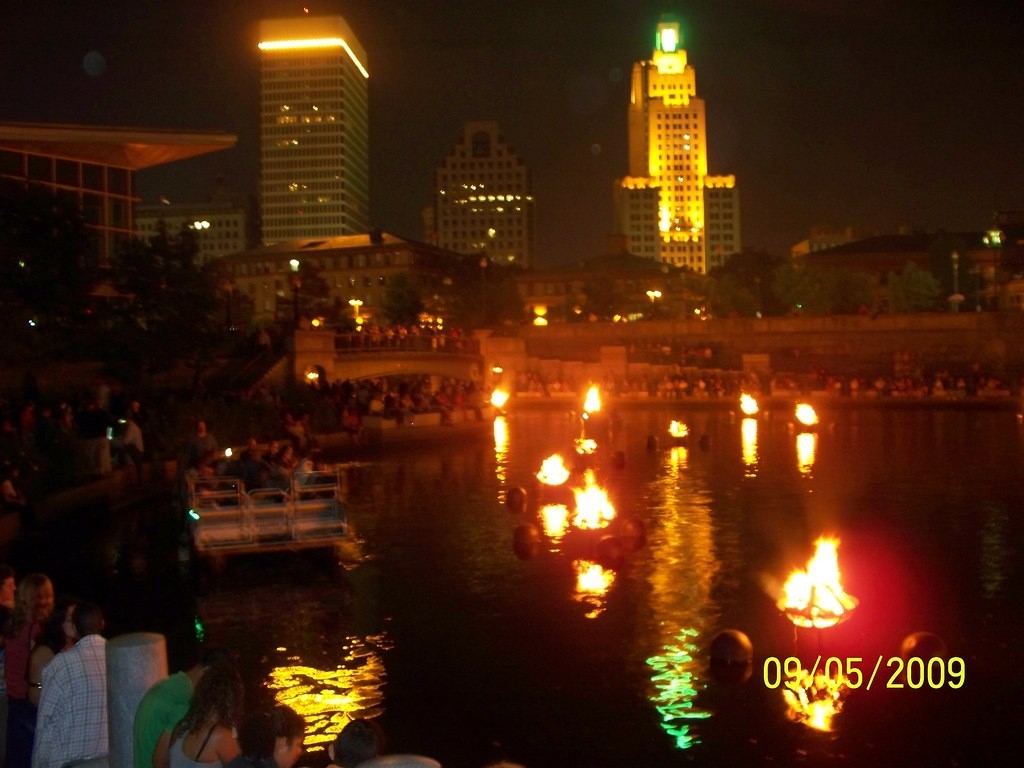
[
  {"left": 0, "top": 305, "right": 1024, "bottom": 518},
  {"left": 0, "top": 565, "right": 376, "bottom": 768}
]
